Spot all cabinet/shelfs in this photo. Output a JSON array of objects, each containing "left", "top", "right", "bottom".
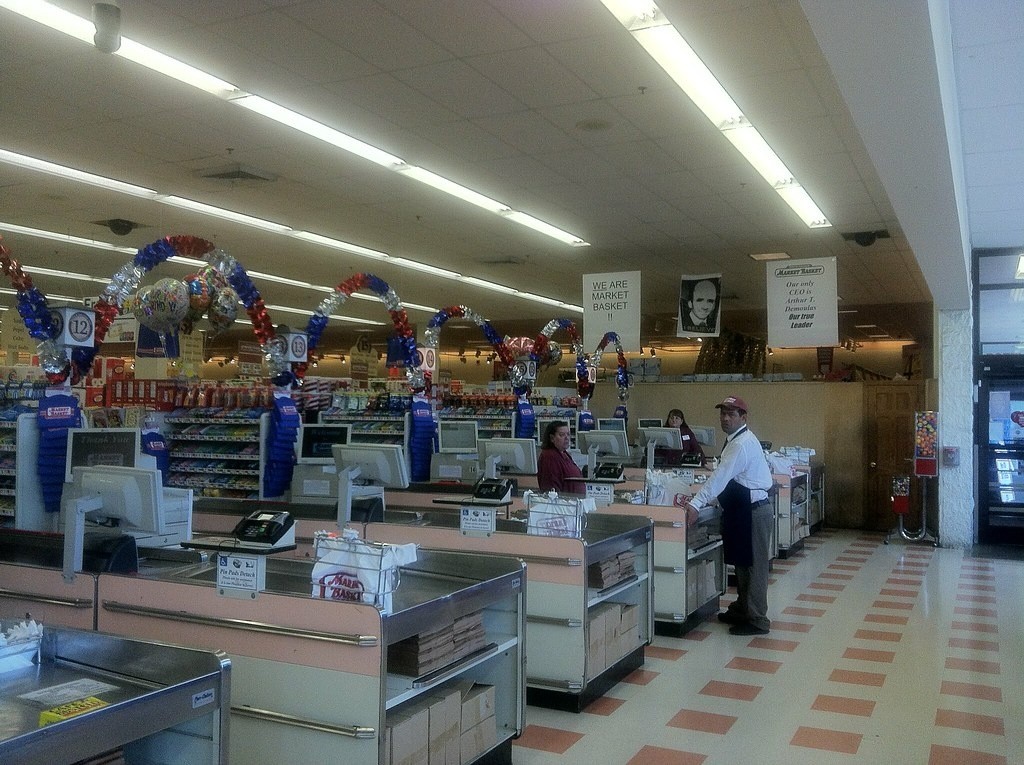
[{"left": 0, "top": 376, "right": 583, "bottom": 517}]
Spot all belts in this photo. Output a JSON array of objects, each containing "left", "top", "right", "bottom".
[{"left": 751, "top": 498, "right": 768, "bottom": 509}]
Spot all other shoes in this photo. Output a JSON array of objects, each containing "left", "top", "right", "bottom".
[
  {"left": 717, "top": 602, "right": 748, "bottom": 624},
  {"left": 729, "top": 616, "right": 770, "bottom": 634}
]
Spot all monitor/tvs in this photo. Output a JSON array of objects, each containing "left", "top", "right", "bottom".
[
  {"left": 72, "top": 466, "right": 165, "bottom": 534},
  {"left": 64, "top": 428, "right": 142, "bottom": 482},
  {"left": 330, "top": 442, "right": 409, "bottom": 490},
  {"left": 439, "top": 418, "right": 716, "bottom": 475},
  {"left": 298, "top": 424, "right": 352, "bottom": 464}
]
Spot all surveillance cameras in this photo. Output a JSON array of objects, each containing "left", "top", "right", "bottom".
[
  {"left": 855, "top": 232, "right": 876, "bottom": 247},
  {"left": 109, "top": 219, "right": 134, "bottom": 236}
]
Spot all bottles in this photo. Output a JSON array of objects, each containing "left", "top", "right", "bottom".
[
  {"left": 712, "top": 455, "right": 717, "bottom": 469},
  {"left": 0, "top": 380, "right": 56, "bottom": 399},
  {"left": 435, "top": 388, "right": 578, "bottom": 410}
]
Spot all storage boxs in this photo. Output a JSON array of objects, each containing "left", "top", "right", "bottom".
[
  {"left": 588, "top": 602, "right": 640, "bottom": 681},
  {"left": 386, "top": 676, "right": 498, "bottom": 765},
  {"left": 686, "top": 559, "right": 716, "bottom": 615},
  {"left": 793, "top": 511, "right": 810, "bottom": 543},
  {"left": 627, "top": 358, "right": 803, "bottom": 383},
  {"left": 85, "top": 356, "right": 176, "bottom": 412}
]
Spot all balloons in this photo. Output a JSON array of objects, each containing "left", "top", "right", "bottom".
[
  {"left": 134, "top": 266, "right": 240, "bottom": 336},
  {"left": 545, "top": 342, "right": 563, "bottom": 364}
]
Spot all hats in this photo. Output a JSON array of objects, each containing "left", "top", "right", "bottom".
[{"left": 715, "top": 397, "right": 748, "bottom": 412}]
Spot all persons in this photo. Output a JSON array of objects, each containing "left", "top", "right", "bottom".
[
  {"left": 687, "top": 395, "right": 774, "bottom": 635},
  {"left": 664, "top": 408, "right": 699, "bottom": 454},
  {"left": 537, "top": 421, "right": 587, "bottom": 495}
]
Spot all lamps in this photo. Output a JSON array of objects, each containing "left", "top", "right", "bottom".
[
  {"left": 841, "top": 340, "right": 857, "bottom": 352},
  {"left": 650, "top": 348, "right": 655, "bottom": 357},
  {"left": 640, "top": 346, "right": 644, "bottom": 356},
  {"left": 768, "top": 347, "right": 774, "bottom": 356}
]
